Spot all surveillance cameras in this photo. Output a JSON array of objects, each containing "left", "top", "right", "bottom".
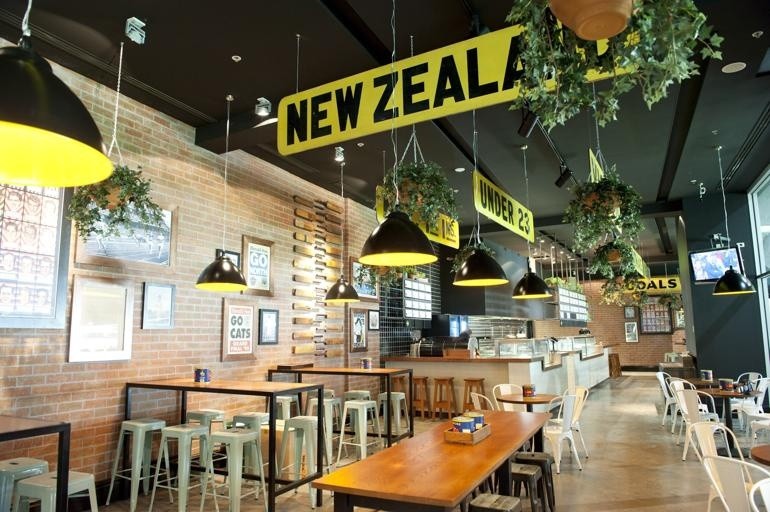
[{"left": 699, "top": 184, "right": 706, "bottom": 200}]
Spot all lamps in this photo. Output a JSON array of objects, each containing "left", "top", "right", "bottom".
[
  {"left": 251, "top": 97, "right": 280, "bottom": 128},
  {"left": 1, "top": 1, "right": 114, "bottom": 188},
  {"left": 711, "top": 144, "right": 757, "bottom": 296},
  {"left": 195, "top": 93, "right": 248, "bottom": 291}
]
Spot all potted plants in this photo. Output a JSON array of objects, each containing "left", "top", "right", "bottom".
[{"left": 64, "top": 165, "right": 170, "bottom": 244}]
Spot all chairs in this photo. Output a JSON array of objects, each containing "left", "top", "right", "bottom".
[
  {"left": 655, "top": 371, "right": 770, "bottom": 512},
  {"left": 543, "top": 395, "right": 584, "bottom": 474},
  {"left": 545, "top": 386, "right": 589, "bottom": 463}
]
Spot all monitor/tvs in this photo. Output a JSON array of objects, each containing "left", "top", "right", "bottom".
[{"left": 688, "top": 245, "right": 746, "bottom": 286}]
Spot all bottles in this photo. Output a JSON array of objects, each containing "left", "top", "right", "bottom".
[{"left": 736, "top": 379, "right": 753, "bottom": 394}]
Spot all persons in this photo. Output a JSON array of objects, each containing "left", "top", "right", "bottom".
[
  {"left": 704, "top": 256, "right": 723, "bottom": 279},
  {"left": 157, "top": 233, "right": 165, "bottom": 259},
  {"left": 97, "top": 226, "right": 105, "bottom": 250},
  {"left": 694, "top": 260, "right": 706, "bottom": 280},
  {"left": 147, "top": 231, "right": 154, "bottom": 254},
  {"left": 1, "top": 192, "right": 56, "bottom": 314},
  {"left": 356, "top": 318, "right": 362, "bottom": 346}
]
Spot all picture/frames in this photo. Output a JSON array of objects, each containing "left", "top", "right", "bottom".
[
  {"left": 350, "top": 308, "right": 369, "bottom": 353},
  {"left": 68, "top": 274, "right": 135, "bottom": 363},
  {"left": 241, "top": 234, "right": 275, "bottom": 297},
  {"left": 74, "top": 197, "right": 180, "bottom": 275},
  {"left": 623, "top": 294, "right": 686, "bottom": 343},
  {"left": 369, "top": 310, "right": 380, "bottom": 331},
  {"left": 221, "top": 298, "right": 259, "bottom": 362},
  {"left": 258, "top": 309, "right": 279, "bottom": 345},
  {"left": 142, "top": 281, "right": 176, "bottom": 330}
]
